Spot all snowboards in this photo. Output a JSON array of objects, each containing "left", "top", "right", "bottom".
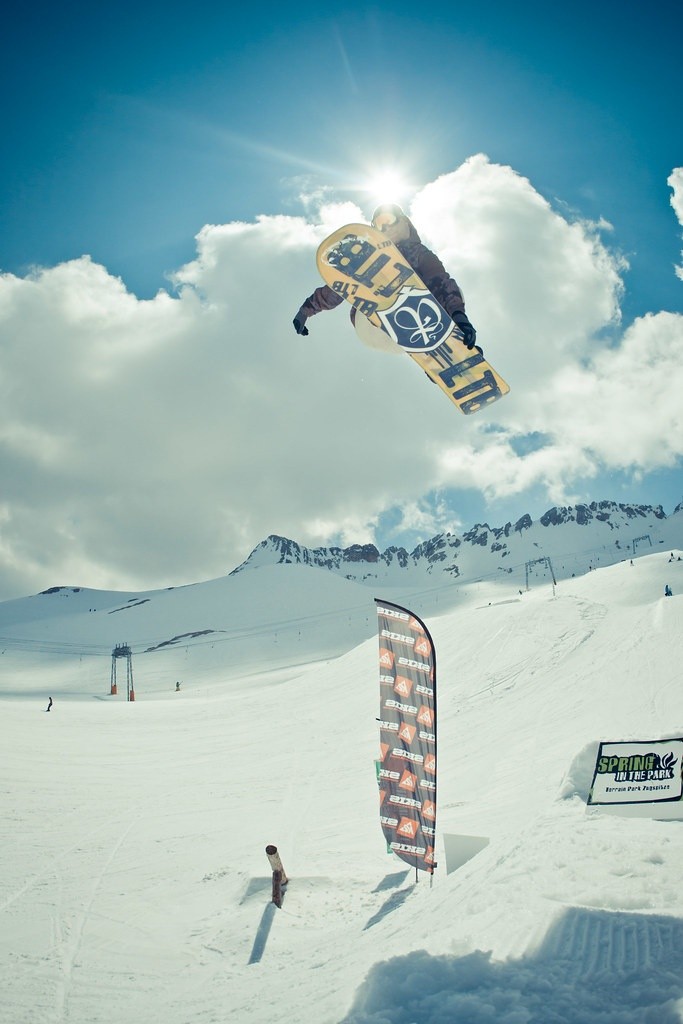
[{"left": 316, "top": 223, "right": 511, "bottom": 417}]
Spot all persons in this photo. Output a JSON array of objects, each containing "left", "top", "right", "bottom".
[
  {"left": 176, "top": 682, "right": 180, "bottom": 691},
  {"left": 665, "top": 585, "right": 672, "bottom": 596},
  {"left": 669, "top": 552, "right": 681, "bottom": 562},
  {"left": 47, "top": 697, "right": 52, "bottom": 711},
  {"left": 293, "top": 201, "right": 483, "bottom": 381}
]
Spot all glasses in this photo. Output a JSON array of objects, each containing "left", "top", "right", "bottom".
[{"left": 371, "top": 213, "right": 397, "bottom": 232}]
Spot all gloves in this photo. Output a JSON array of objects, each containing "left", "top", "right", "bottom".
[
  {"left": 454, "top": 314, "right": 476, "bottom": 350},
  {"left": 293, "top": 312, "right": 308, "bottom": 335}
]
